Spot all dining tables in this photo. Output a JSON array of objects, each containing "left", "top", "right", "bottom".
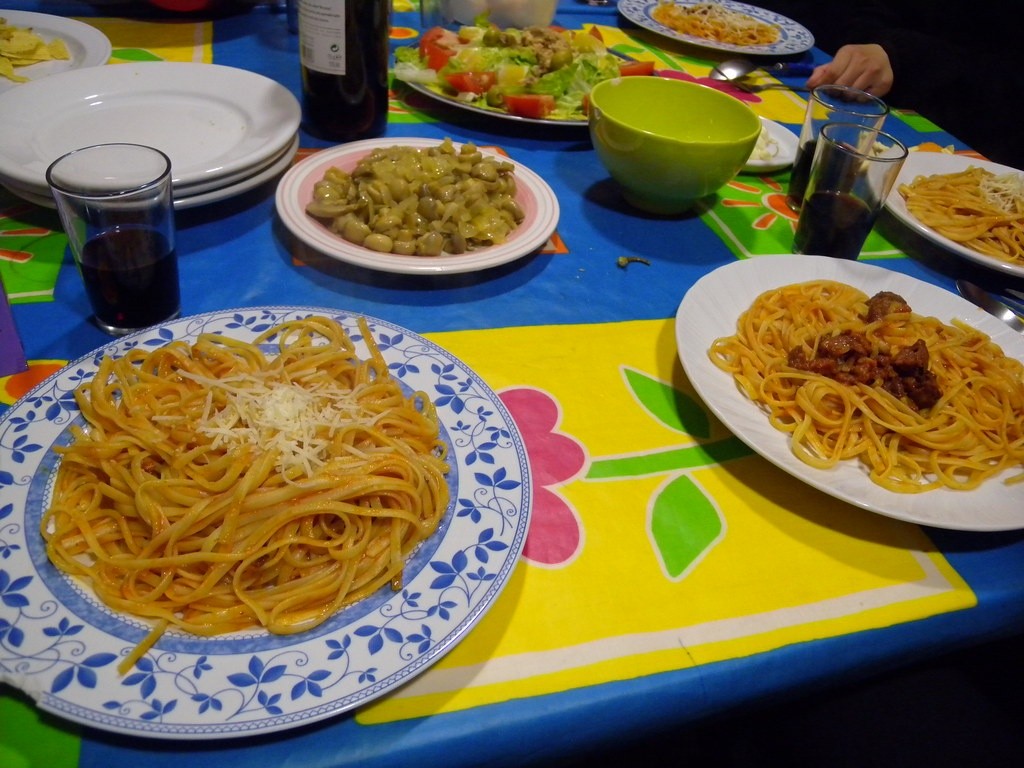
[{"left": 0, "top": 1, "right": 1022, "bottom": 766}]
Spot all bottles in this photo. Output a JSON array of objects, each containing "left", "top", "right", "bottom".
[{"left": 296, "top": 0, "right": 391, "bottom": 142}]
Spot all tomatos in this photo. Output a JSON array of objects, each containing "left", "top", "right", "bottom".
[
  {"left": 619, "top": 59, "right": 654, "bottom": 77},
  {"left": 445, "top": 70, "right": 496, "bottom": 93},
  {"left": 501, "top": 93, "right": 555, "bottom": 119},
  {"left": 426, "top": 44, "right": 454, "bottom": 72},
  {"left": 419, "top": 26, "right": 467, "bottom": 58}
]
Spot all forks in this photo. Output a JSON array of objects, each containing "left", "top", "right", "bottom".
[{"left": 712, "top": 65, "right": 812, "bottom": 94}]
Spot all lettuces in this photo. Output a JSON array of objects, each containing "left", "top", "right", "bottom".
[{"left": 390, "top": 12, "right": 625, "bottom": 118}]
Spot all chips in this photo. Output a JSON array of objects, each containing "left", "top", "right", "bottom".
[{"left": 0, "top": 15, "right": 70, "bottom": 82}]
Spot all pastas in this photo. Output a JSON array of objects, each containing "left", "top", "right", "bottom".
[
  {"left": 896, "top": 163, "right": 1024, "bottom": 267},
  {"left": 710, "top": 281, "right": 1024, "bottom": 495},
  {"left": 654, "top": 0, "right": 778, "bottom": 46},
  {"left": 40, "top": 313, "right": 449, "bottom": 676}
]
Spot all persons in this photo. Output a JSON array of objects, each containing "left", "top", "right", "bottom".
[{"left": 807, "top": 0, "right": 1024, "bottom": 171}]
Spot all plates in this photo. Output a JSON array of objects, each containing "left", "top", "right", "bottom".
[
  {"left": 866, "top": 151, "right": 1024, "bottom": 277},
  {"left": 674, "top": 253, "right": 1024, "bottom": 531},
  {"left": 273, "top": 137, "right": 559, "bottom": 276},
  {"left": 0, "top": 61, "right": 301, "bottom": 211},
  {"left": 394, "top": 30, "right": 659, "bottom": 126},
  {"left": 0, "top": 9, "right": 112, "bottom": 95},
  {"left": 0, "top": 305, "right": 534, "bottom": 741},
  {"left": 616, "top": 0, "right": 815, "bottom": 55},
  {"left": 740, "top": 115, "right": 800, "bottom": 172}
]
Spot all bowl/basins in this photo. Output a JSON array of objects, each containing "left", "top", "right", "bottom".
[{"left": 588, "top": 75, "right": 763, "bottom": 214}]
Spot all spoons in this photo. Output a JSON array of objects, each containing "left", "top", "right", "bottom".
[{"left": 708, "top": 58, "right": 823, "bottom": 81}]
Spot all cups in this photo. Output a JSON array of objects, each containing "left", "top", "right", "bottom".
[
  {"left": 44, "top": 143, "right": 181, "bottom": 338},
  {"left": 784, "top": 84, "right": 891, "bottom": 214},
  {"left": 791, "top": 122, "right": 909, "bottom": 260}
]
